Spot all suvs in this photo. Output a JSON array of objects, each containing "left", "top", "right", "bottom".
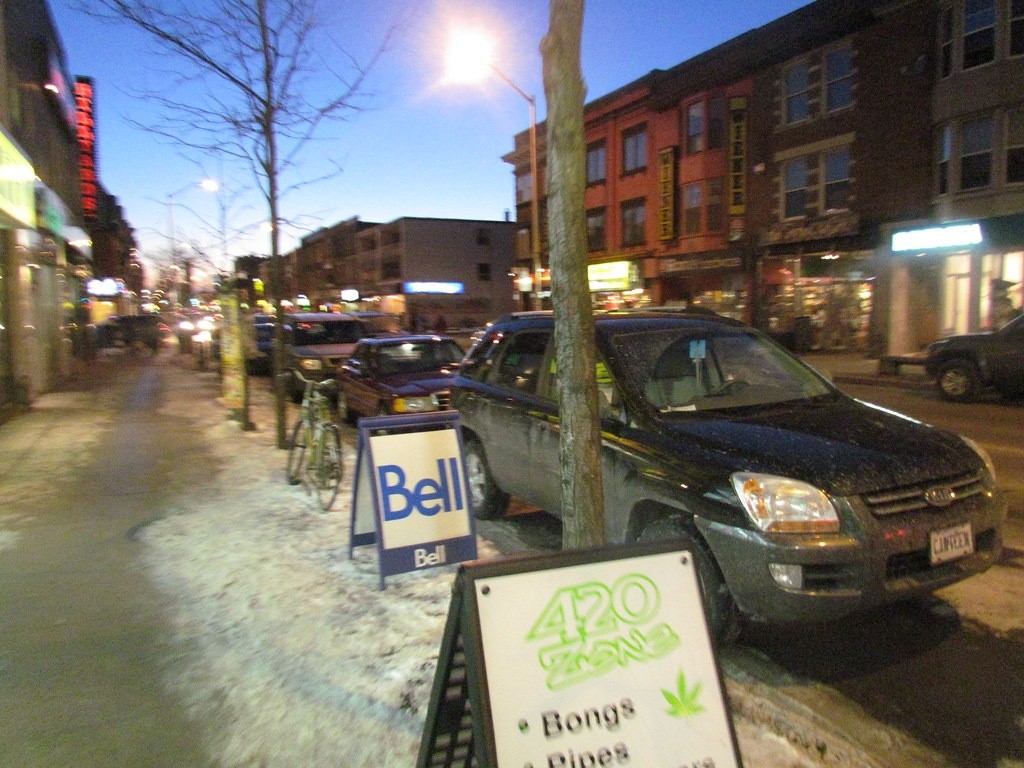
[
  {"left": 351, "top": 313, "right": 413, "bottom": 339},
  {"left": 272, "top": 313, "right": 367, "bottom": 400},
  {"left": 451, "top": 309, "right": 1006, "bottom": 653}
]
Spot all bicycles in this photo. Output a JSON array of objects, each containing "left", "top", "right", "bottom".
[{"left": 280, "top": 368, "right": 345, "bottom": 512}]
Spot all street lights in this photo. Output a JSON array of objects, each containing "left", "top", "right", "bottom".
[
  {"left": 444, "top": 27, "right": 542, "bottom": 313},
  {"left": 167, "top": 179, "right": 220, "bottom": 297}
]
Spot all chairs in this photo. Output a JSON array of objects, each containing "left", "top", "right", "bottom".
[
  {"left": 415, "top": 350, "right": 441, "bottom": 370},
  {"left": 379, "top": 353, "right": 404, "bottom": 377},
  {"left": 592, "top": 352, "right": 710, "bottom": 418}
]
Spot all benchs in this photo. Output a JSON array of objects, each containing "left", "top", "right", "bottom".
[{"left": 879, "top": 354, "right": 927, "bottom": 374}]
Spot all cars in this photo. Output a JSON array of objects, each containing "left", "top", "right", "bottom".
[
  {"left": 174, "top": 308, "right": 276, "bottom": 357},
  {"left": 94, "top": 316, "right": 169, "bottom": 353},
  {"left": 247, "top": 323, "right": 275, "bottom": 373},
  {"left": 924, "top": 312, "right": 1024, "bottom": 404},
  {"left": 334, "top": 337, "right": 466, "bottom": 435}
]
